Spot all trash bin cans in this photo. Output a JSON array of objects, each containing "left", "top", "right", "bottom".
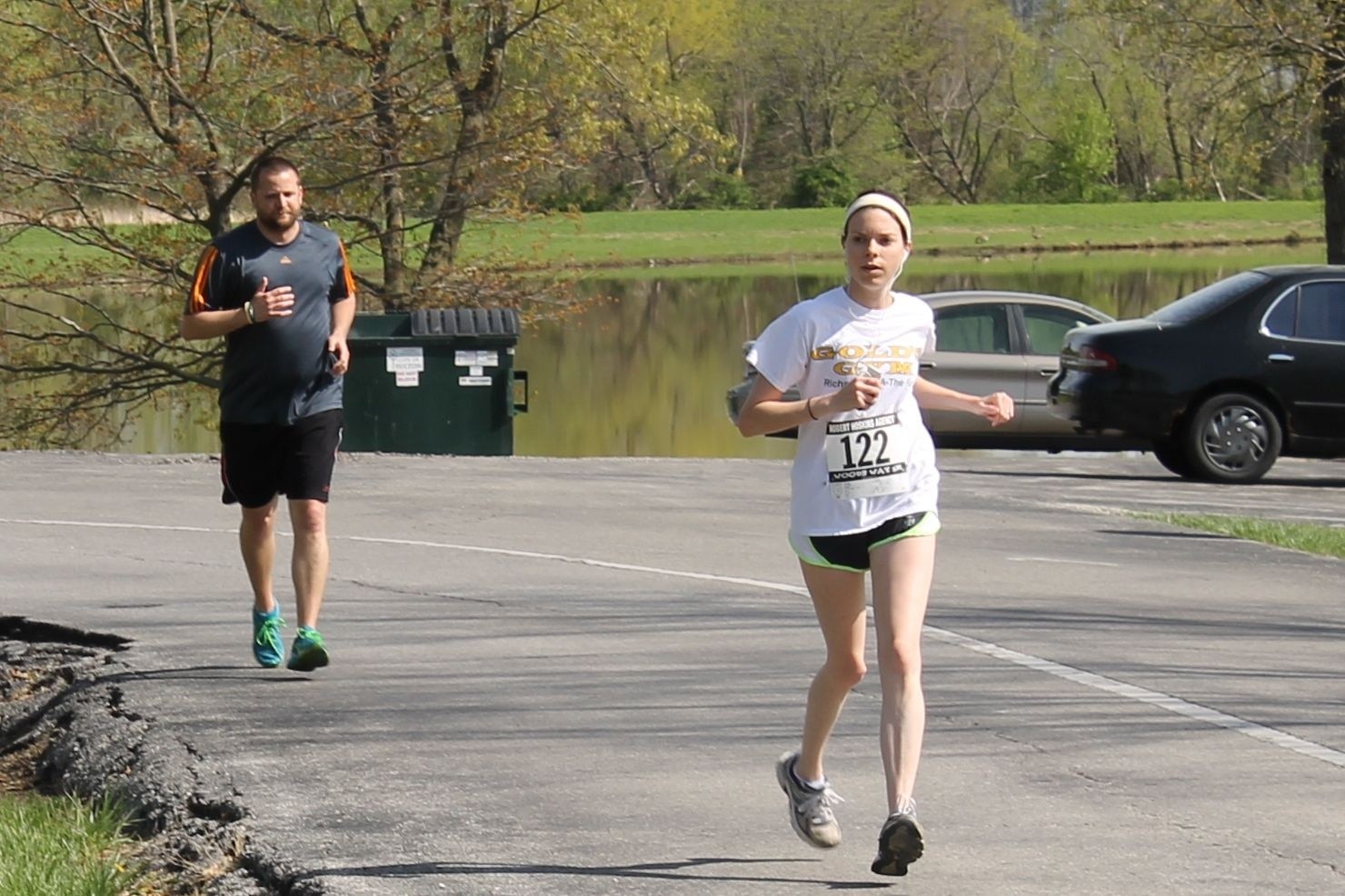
[{"left": 334, "top": 307, "right": 530, "bottom": 455}]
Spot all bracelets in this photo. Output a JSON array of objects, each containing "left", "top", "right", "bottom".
[
  {"left": 245, "top": 300, "right": 256, "bottom": 325},
  {"left": 807, "top": 396, "right": 820, "bottom": 420}
]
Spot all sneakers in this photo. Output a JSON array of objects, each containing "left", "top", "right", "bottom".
[
  {"left": 285, "top": 625, "right": 329, "bottom": 672},
  {"left": 252, "top": 597, "right": 288, "bottom": 666},
  {"left": 871, "top": 814, "right": 925, "bottom": 876},
  {"left": 776, "top": 751, "right": 846, "bottom": 849}
]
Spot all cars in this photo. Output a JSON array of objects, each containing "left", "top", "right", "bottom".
[
  {"left": 724, "top": 289, "right": 1153, "bottom": 449},
  {"left": 1044, "top": 264, "right": 1344, "bottom": 483}
]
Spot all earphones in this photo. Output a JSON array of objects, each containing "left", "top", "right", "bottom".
[{"left": 902, "top": 249, "right": 910, "bottom": 265}]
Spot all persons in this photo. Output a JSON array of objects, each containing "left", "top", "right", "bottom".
[
  {"left": 737, "top": 189, "right": 1014, "bottom": 876},
  {"left": 180, "top": 159, "right": 356, "bottom": 672}
]
